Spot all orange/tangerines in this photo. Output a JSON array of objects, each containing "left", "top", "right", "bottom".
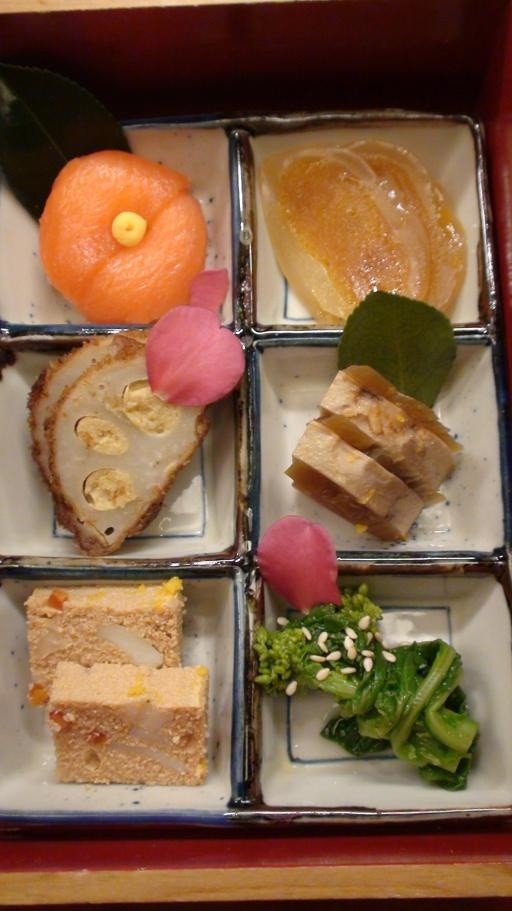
[{"left": 40, "top": 151, "right": 207, "bottom": 324}]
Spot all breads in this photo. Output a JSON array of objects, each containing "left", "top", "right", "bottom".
[
  {"left": 287, "top": 369, "right": 464, "bottom": 540},
  {"left": 24, "top": 579, "right": 182, "bottom": 707},
  {"left": 29, "top": 330, "right": 216, "bottom": 557},
  {"left": 49, "top": 662, "right": 209, "bottom": 786}
]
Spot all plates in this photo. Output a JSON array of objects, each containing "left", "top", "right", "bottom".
[
  {"left": 2, "top": 561, "right": 243, "bottom": 824},
  {"left": 236, "top": 112, "right": 504, "bottom": 340},
  {"left": 2, "top": 111, "right": 232, "bottom": 327},
  {"left": 3, "top": 328, "right": 243, "bottom": 566},
  {"left": 251, "top": 560, "right": 512, "bottom": 830},
  {"left": 245, "top": 334, "right": 509, "bottom": 565}
]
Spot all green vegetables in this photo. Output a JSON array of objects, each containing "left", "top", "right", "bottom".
[{"left": 245, "top": 584, "right": 479, "bottom": 793}]
